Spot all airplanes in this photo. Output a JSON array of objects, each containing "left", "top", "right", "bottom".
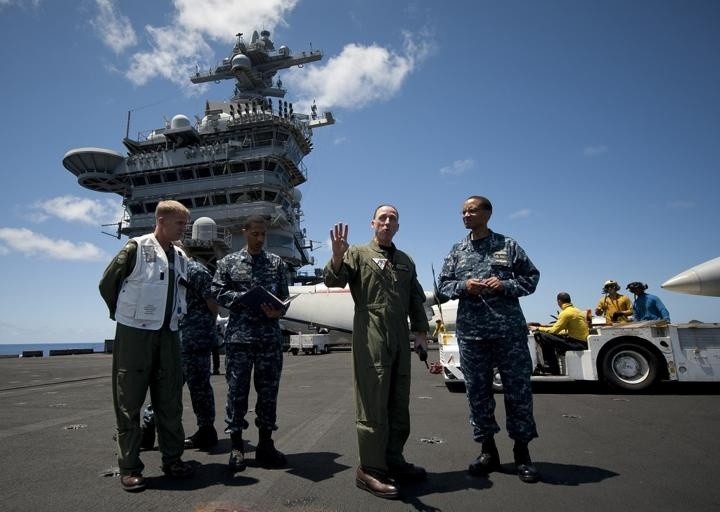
[
  {"left": 659, "top": 254, "right": 720, "bottom": 298},
  {"left": 214, "top": 263, "right": 451, "bottom": 340}
]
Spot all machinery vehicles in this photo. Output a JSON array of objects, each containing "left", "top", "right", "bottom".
[
  {"left": 436, "top": 313, "right": 720, "bottom": 392},
  {"left": 287, "top": 331, "right": 331, "bottom": 354}
]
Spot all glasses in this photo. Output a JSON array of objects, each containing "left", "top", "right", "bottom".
[{"left": 606, "top": 285, "right": 614, "bottom": 288}]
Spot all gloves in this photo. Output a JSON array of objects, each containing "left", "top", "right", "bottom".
[{"left": 413, "top": 333, "right": 427, "bottom": 353}]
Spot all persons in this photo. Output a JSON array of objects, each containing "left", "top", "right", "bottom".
[
  {"left": 594, "top": 280, "right": 633, "bottom": 326},
  {"left": 322, "top": 204, "right": 430, "bottom": 498},
  {"left": 211, "top": 216, "right": 290, "bottom": 472},
  {"left": 112, "top": 240, "right": 219, "bottom": 450},
  {"left": 212, "top": 323, "right": 220, "bottom": 375},
  {"left": 437, "top": 196, "right": 540, "bottom": 482},
  {"left": 99, "top": 200, "right": 202, "bottom": 490},
  {"left": 432, "top": 320, "right": 447, "bottom": 337},
  {"left": 626, "top": 282, "right": 670, "bottom": 324},
  {"left": 528, "top": 292, "right": 590, "bottom": 376}
]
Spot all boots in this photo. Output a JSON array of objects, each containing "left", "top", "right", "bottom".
[
  {"left": 513, "top": 440, "right": 538, "bottom": 482},
  {"left": 255, "top": 428, "right": 288, "bottom": 467},
  {"left": 113, "top": 423, "right": 155, "bottom": 449},
  {"left": 469, "top": 438, "right": 501, "bottom": 476},
  {"left": 228, "top": 430, "right": 246, "bottom": 471},
  {"left": 184, "top": 424, "right": 218, "bottom": 449}
]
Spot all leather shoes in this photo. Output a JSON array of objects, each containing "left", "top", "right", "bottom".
[
  {"left": 162, "top": 459, "right": 193, "bottom": 476},
  {"left": 390, "top": 460, "right": 428, "bottom": 482},
  {"left": 119, "top": 473, "right": 147, "bottom": 490},
  {"left": 356, "top": 463, "right": 402, "bottom": 498}
]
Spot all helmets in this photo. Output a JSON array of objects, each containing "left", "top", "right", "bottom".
[
  {"left": 627, "top": 282, "right": 642, "bottom": 292},
  {"left": 604, "top": 280, "right": 617, "bottom": 290}
]
